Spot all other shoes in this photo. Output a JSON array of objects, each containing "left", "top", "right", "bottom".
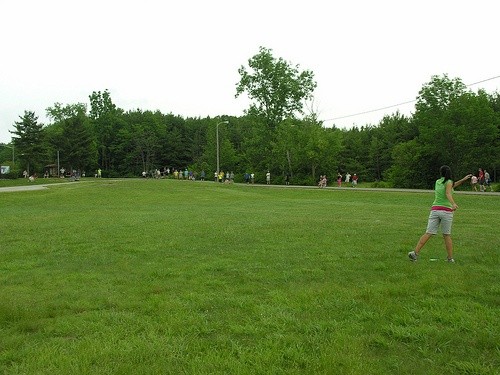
[
  {"left": 408, "top": 251, "right": 417, "bottom": 260},
  {"left": 447, "top": 258, "right": 454, "bottom": 263}
]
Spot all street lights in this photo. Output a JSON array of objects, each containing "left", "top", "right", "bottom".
[{"left": 217, "top": 120, "right": 230, "bottom": 182}]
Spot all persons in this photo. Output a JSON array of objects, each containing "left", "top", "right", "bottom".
[
  {"left": 23, "top": 170, "right": 28, "bottom": 178},
  {"left": 44, "top": 171, "right": 49, "bottom": 178},
  {"left": 81, "top": 169, "right": 85, "bottom": 176},
  {"left": 29, "top": 173, "right": 37, "bottom": 181},
  {"left": 72, "top": 170, "right": 79, "bottom": 181},
  {"left": 408, "top": 165, "right": 472, "bottom": 263},
  {"left": 174, "top": 169, "right": 192, "bottom": 180},
  {"left": 266, "top": 171, "right": 270, "bottom": 184},
  {"left": 60, "top": 167, "right": 65, "bottom": 175},
  {"left": 484, "top": 170, "right": 491, "bottom": 187},
  {"left": 201, "top": 170, "right": 205, "bottom": 181},
  {"left": 478, "top": 168, "right": 486, "bottom": 191},
  {"left": 157, "top": 169, "right": 160, "bottom": 177},
  {"left": 337, "top": 173, "right": 342, "bottom": 187},
  {"left": 345, "top": 172, "right": 351, "bottom": 182},
  {"left": 471, "top": 173, "right": 478, "bottom": 191},
  {"left": 285, "top": 172, "right": 289, "bottom": 186},
  {"left": 245, "top": 172, "right": 254, "bottom": 184},
  {"left": 215, "top": 170, "right": 234, "bottom": 182},
  {"left": 351, "top": 174, "right": 358, "bottom": 184},
  {"left": 142, "top": 171, "right": 146, "bottom": 177},
  {"left": 320, "top": 175, "right": 326, "bottom": 186},
  {"left": 98, "top": 168, "right": 102, "bottom": 178},
  {"left": 164, "top": 168, "right": 170, "bottom": 175}
]
